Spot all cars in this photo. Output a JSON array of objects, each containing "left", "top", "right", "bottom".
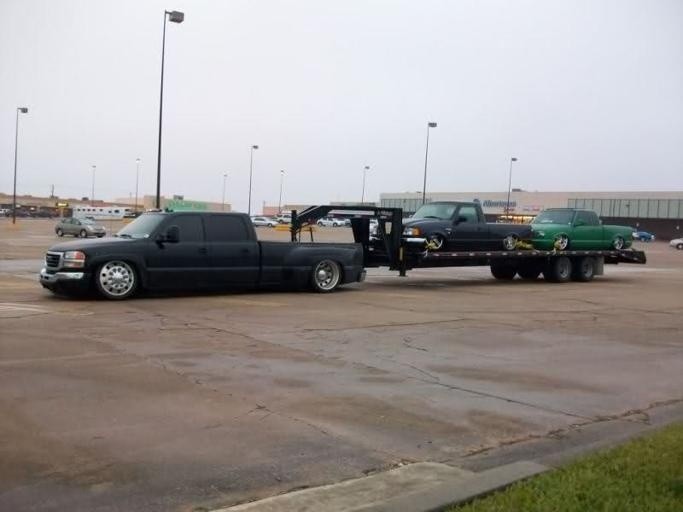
[
  {"left": 53, "top": 214, "right": 107, "bottom": 239},
  {"left": 633, "top": 231, "right": 655, "bottom": 243},
  {"left": 668, "top": 238, "right": 683, "bottom": 250},
  {"left": 0, "top": 203, "right": 72, "bottom": 219},
  {"left": 247, "top": 209, "right": 379, "bottom": 228}
]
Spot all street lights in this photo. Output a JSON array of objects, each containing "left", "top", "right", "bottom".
[
  {"left": 135, "top": 158, "right": 140, "bottom": 212},
  {"left": 155, "top": 9, "right": 185, "bottom": 212},
  {"left": 91, "top": 165, "right": 96, "bottom": 207},
  {"left": 505, "top": 157, "right": 519, "bottom": 223},
  {"left": 277, "top": 169, "right": 285, "bottom": 213},
  {"left": 421, "top": 122, "right": 437, "bottom": 206},
  {"left": 247, "top": 145, "right": 258, "bottom": 216},
  {"left": 361, "top": 166, "right": 369, "bottom": 206},
  {"left": 11, "top": 107, "right": 28, "bottom": 224},
  {"left": 221, "top": 173, "right": 227, "bottom": 210}
]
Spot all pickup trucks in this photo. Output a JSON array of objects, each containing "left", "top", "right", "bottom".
[
  {"left": 368, "top": 199, "right": 536, "bottom": 256},
  {"left": 522, "top": 204, "right": 636, "bottom": 253},
  {"left": 36, "top": 208, "right": 369, "bottom": 301}
]
